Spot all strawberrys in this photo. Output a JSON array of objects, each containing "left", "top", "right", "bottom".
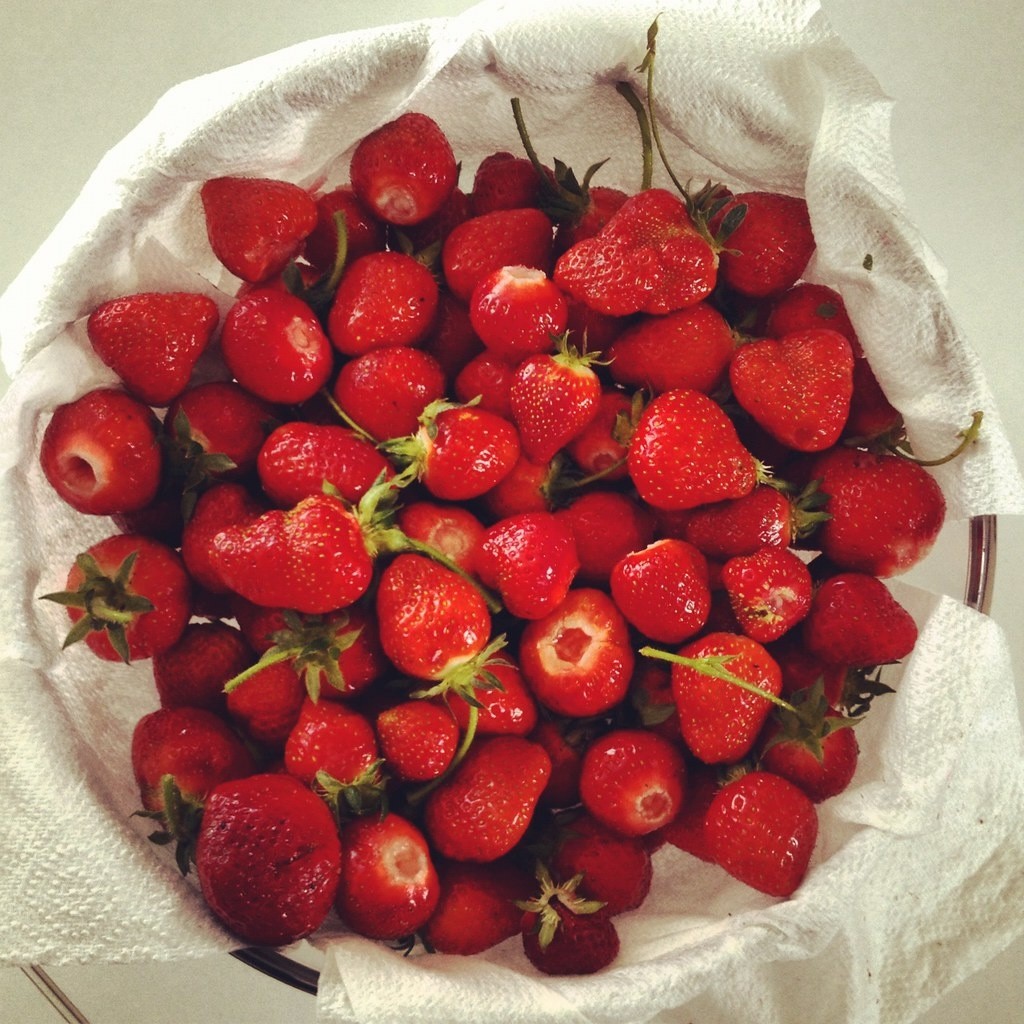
[{"left": 42, "top": 88, "right": 946, "bottom": 970}]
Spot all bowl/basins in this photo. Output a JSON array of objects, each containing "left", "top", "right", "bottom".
[{"left": 1, "top": 15, "right": 1001, "bottom": 1013}]
